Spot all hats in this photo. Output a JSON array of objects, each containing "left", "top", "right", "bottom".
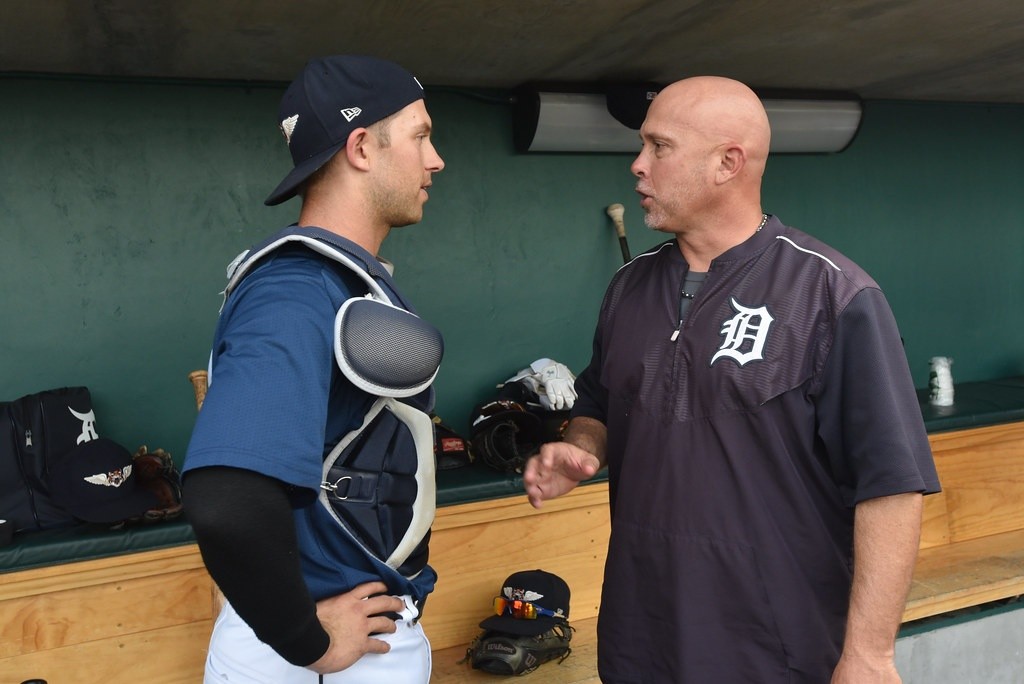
[
  {"left": 479, "top": 569, "right": 570, "bottom": 635},
  {"left": 53, "top": 439, "right": 150, "bottom": 523},
  {"left": 264, "top": 53, "right": 426, "bottom": 205}
]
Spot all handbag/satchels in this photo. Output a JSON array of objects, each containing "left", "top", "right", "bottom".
[{"left": 0, "top": 387, "right": 100, "bottom": 544}]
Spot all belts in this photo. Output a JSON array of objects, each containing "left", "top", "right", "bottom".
[{"left": 371, "top": 596, "right": 426, "bottom": 625}]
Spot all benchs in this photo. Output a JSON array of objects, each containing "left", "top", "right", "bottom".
[{"left": 0, "top": 373, "right": 1024, "bottom": 684}]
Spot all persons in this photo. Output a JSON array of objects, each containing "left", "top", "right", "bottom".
[
  {"left": 524, "top": 74, "right": 940, "bottom": 684},
  {"left": 179, "top": 54, "right": 444, "bottom": 684}
]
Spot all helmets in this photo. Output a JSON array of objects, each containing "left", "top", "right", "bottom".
[
  {"left": 470, "top": 400, "right": 548, "bottom": 480},
  {"left": 133, "top": 455, "right": 183, "bottom": 520}
]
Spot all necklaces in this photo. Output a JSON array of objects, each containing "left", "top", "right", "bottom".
[{"left": 680, "top": 213, "right": 770, "bottom": 299}]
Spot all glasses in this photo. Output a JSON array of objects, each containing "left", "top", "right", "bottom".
[{"left": 493, "top": 596, "right": 566, "bottom": 619}]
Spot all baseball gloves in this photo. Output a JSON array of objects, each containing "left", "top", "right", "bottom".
[
  {"left": 111, "top": 444, "right": 186, "bottom": 533},
  {"left": 454, "top": 621, "right": 577, "bottom": 677}
]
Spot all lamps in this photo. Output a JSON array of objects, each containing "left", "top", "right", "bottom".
[{"left": 513, "top": 86, "right": 865, "bottom": 157}]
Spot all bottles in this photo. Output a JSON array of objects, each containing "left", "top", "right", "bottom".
[{"left": 929, "top": 356, "right": 953, "bottom": 409}]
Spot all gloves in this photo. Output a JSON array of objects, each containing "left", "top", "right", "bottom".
[{"left": 505, "top": 357, "right": 579, "bottom": 413}]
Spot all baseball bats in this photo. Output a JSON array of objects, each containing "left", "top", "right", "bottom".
[
  {"left": 187, "top": 369, "right": 209, "bottom": 411},
  {"left": 605, "top": 201, "right": 635, "bottom": 261}
]
